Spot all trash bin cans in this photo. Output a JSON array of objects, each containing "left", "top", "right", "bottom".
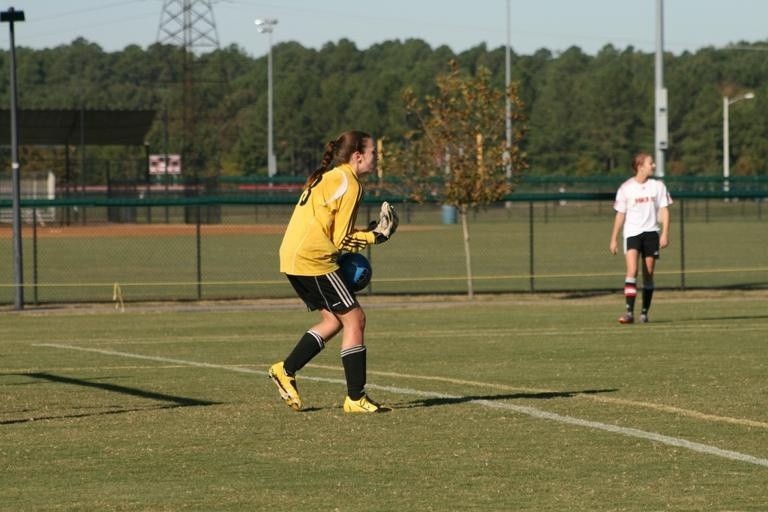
[{"left": 441, "top": 205, "right": 456, "bottom": 224}]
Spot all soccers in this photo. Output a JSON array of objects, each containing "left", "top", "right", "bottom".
[{"left": 337, "top": 253, "right": 371, "bottom": 290}]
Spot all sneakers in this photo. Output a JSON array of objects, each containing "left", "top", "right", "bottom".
[
  {"left": 616, "top": 312, "right": 634, "bottom": 325},
  {"left": 341, "top": 389, "right": 392, "bottom": 415},
  {"left": 636, "top": 313, "right": 649, "bottom": 324},
  {"left": 266, "top": 360, "right": 305, "bottom": 413}
]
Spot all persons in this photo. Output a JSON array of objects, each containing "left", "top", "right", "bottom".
[
  {"left": 609, "top": 153, "right": 675, "bottom": 325},
  {"left": 268, "top": 131, "right": 399, "bottom": 413}
]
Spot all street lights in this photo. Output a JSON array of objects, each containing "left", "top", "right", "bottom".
[
  {"left": 722, "top": 92, "right": 755, "bottom": 202},
  {"left": 252, "top": 17, "right": 282, "bottom": 190},
  {"left": 0, "top": 7, "right": 32, "bottom": 310}
]
